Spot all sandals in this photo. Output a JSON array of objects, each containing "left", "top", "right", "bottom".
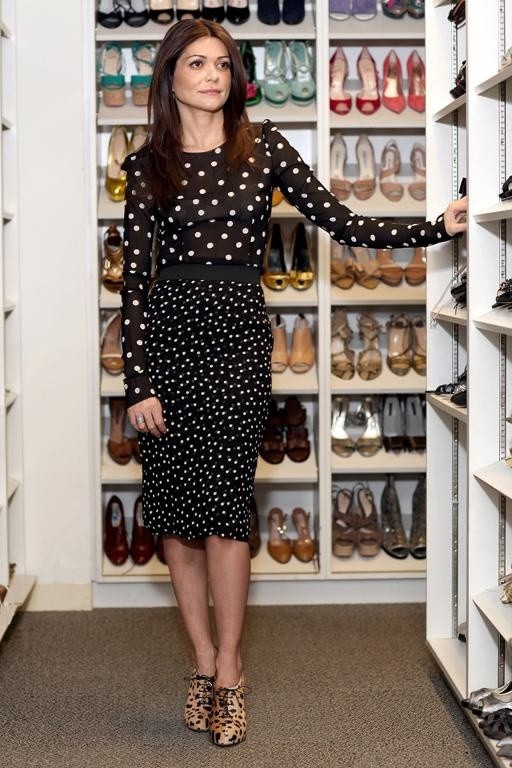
[
  {"left": 331, "top": 394, "right": 426, "bottom": 458},
  {"left": 435, "top": 366, "right": 466, "bottom": 405},
  {"left": 332, "top": 475, "right": 426, "bottom": 558},
  {"left": 331, "top": 306, "right": 426, "bottom": 381},
  {"left": 258, "top": 396, "right": 310, "bottom": 463},
  {"left": 492, "top": 279, "right": 512, "bottom": 309},
  {"left": 461, "top": 681, "right": 512, "bottom": 760},
  {"left": 449, "top": 61, "right": 466, "bottom": 98},
  {"left": 448, "top": 0, "right": 466, "bottom": 29},
  {"left": 497, "top": 46, "right": 511, "bottom": 72},
  {"left": 328, "top": 1, "right": 425, "bottom": 20},
  {"left": 331, "top": 237, "right": 426, "bottom": 290},
  {"left": 107, "top": 397, "right": 143, "bottom": 465},
  {"left": 270, "top": 313, "right": 316, "bottom": 373},
  {"left": 499, "top": 175, "right": 512, "bottom": 202},
  {"left": 96, "top": 1, "right": 317, "bottom": 107},
  {"left": 101, "top": 224, "right": 124, "bottom": 293},
  {"left": 500, "top": 581, "right": 512, "bottom": 604},
  {"left": 100, "top": 308, "right": 125, "bottom": 375},
  {"left": 451, "top": 274, "right": 466, "bottom": 303},
  {"left": 330, "top": 132, "right": 426, "bottom": 201}
]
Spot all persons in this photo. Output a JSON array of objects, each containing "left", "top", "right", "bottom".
[{"left": 119, "top": 18, "right": 467, "bottom": 747}]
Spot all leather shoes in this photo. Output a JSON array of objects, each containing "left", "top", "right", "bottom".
[
  {"left": 330, "top": 47, "right": 426, "bottom": 115},
  {"left": 106, "top": 126, "right": 149, "bottom": 202},
  {"left": 267, "top": 506, "right": 317, "bottom": 564},
  {"left": 262, "top": 221, "right": 315, "bottom": 291},
  {"left": 131, "top": 495, "right": 168, "bottom": 565},
  {"left": 104, "top": 495, "right": 128, "bottom": 565},
  {"left": 184, "top": 646, "right": 252, "bottom": 746},
  {"left": 272, "top": 188, "right": 285, "bottom": 207}
]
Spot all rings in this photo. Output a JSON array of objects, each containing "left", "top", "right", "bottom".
[{"left": 136, "top": 415, "right": 144, "bottom": 424}]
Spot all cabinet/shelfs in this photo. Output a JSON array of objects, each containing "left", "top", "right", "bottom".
[
  {"left": 0, "top": 20, "right": 37, "bottom": 640},
  {"left": 424, "top": 2, "right": 512, "bottom": 768},
  {"left": 84, "top": 0, "right": 424, "bottom": 587}
]
